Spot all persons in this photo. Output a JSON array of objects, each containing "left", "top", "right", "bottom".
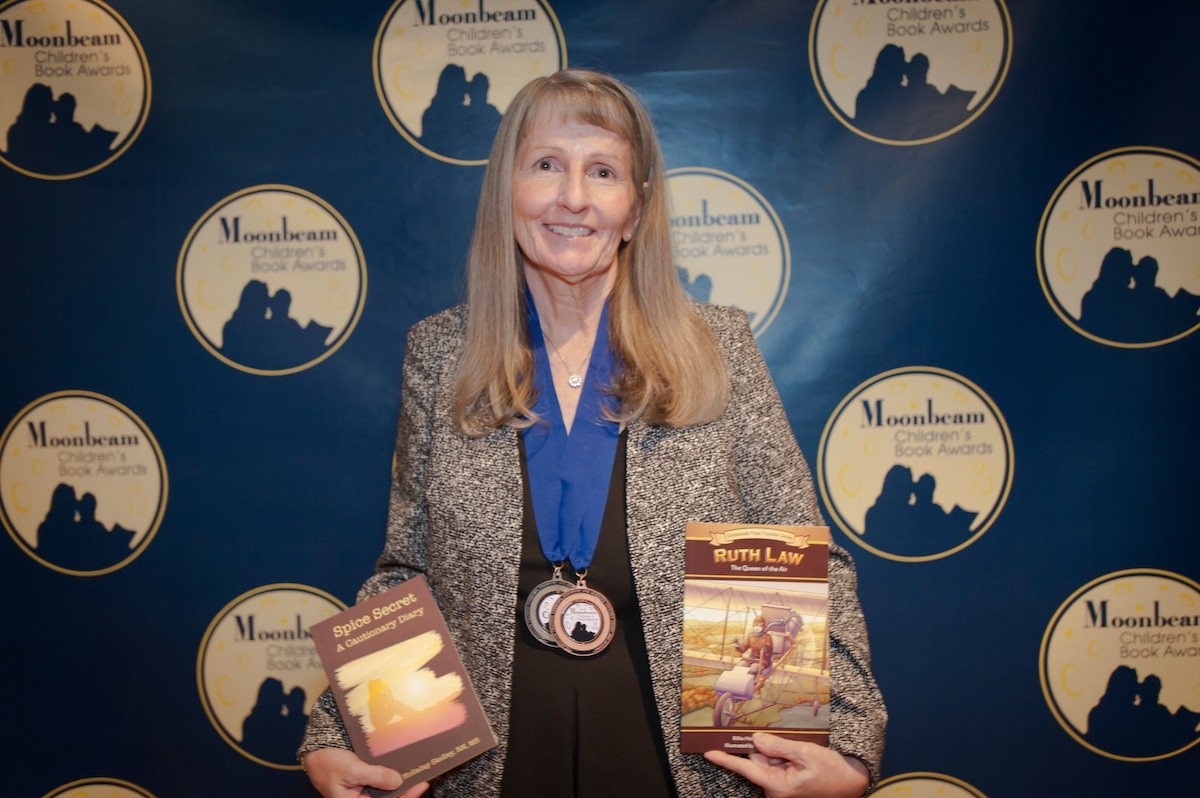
[{"left": 297, "top": 69, "right": 887, "bottom": 798}]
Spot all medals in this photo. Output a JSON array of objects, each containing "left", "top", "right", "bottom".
[{"left": 524, "top": 572, "right": 616, "bottom": 656}]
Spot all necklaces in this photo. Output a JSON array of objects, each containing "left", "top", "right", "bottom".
[{"left": 542, "top": 330, "right": 594, "bottom": 388}]
